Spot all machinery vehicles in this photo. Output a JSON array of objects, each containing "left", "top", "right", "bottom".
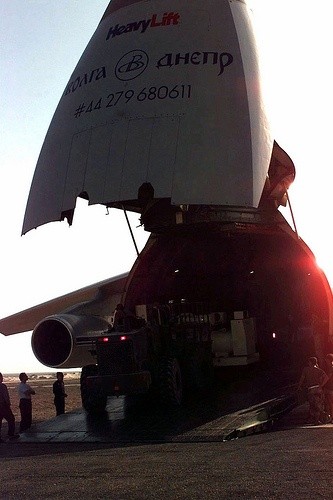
[{"left": 80, "top": 304, "right": 213, "bottom": 417}]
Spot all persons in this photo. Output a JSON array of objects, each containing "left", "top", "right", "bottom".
[
  {"left": 53, "top": 372, "right": 68, "bottom": 416},
  {"left": 18, "top": 373, "right": 36, "bottom": 431},
  {"left": 297, "top": 356, "right": 329, "bottom": 424},
  {"left": 0, "top": 373, "right": 20, "bottom": 440},
  {"left": 323, "top": 353, "right": 333, "bottom": 424}
]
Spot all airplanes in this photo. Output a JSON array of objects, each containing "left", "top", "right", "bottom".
[{"left": 0, "top": 0, "right": 333, "bottom": 442}]
